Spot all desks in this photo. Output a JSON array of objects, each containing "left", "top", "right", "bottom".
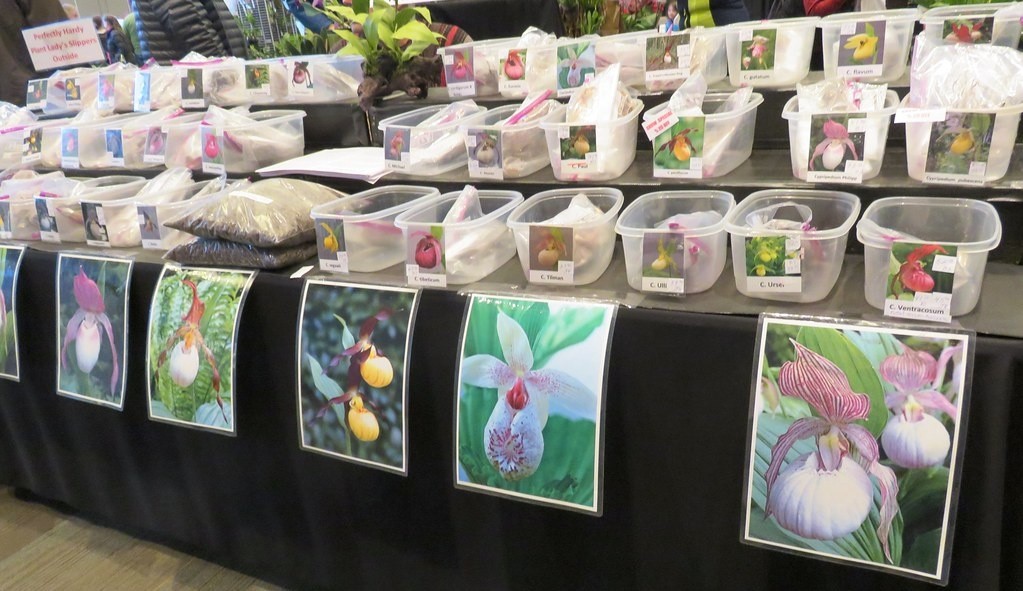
[{"left": 0, "top": 247, "right": 1023, "bottom": 590}]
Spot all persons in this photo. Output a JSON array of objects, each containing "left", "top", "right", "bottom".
[
  {"left": 0, "top": 0, "right": 248, "bottom": 108},
  {"left": 284, "top": 0, "right": 564, "bottom": 47},
  {"left": 664, "top": 0, "right": 911, "bottom": 33}
]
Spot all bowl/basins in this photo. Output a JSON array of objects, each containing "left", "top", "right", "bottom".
[
  {"left": 0, "top": 110, "right": 307, "bottom": 177},
  {"left": 394, "top": 189, "right": 525, "bottom": 284},
  {"left": 643, "top": 93, "right": 763, "bottom": 177},
  {"left": 310, "top": 185, "right": 440, "bottom": 272},
  {"left": 781, "top": 89, "right": 899, "bottom": 180},
  {"left": 856, "top": 196, "right": 1003, "bottom": 316},
  {"left": 379, "top": 99, "right": 645, "bottom": 181},
  {"left": 901, "top": 91, "right": 1021, "bottom": 184},
  {"left": 0, "top": 176, "right": 246, "bottom": 250},
  {"left": 436, "top": 4, "right": 1023, "bottom": 101},
  {"left": 41, "top": 55, "right": 367, "bottom": 115},
  {"left": 614, "top": 190, "right": 736, "bottom": 294},
  {"left": 721, "top": 189, "right": 861, "bottom": 302},
  {"left": 507, "top": 188, "right": 624, "bottom": 285}
]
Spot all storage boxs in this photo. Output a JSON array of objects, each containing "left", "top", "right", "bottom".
[{"left": 0, "top": 1, "right": 1023, "bottom": 318}]
